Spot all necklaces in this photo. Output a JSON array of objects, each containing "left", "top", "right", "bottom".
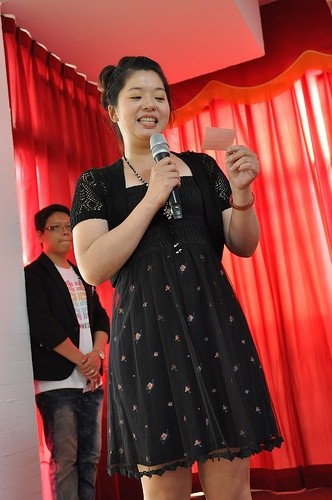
[{"left": 122, "top": 151, "right": 173, "bottom": 219}]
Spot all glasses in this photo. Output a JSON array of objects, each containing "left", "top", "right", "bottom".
[{"left": 42, "top": 222, "right": 72, "bottom": 232}]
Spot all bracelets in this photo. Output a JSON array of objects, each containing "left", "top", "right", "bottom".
[{"left": 230, "top": 191, "right": 255, "bottom": 210}]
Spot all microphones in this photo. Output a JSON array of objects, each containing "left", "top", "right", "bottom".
[{"left": 150, "top": 133, "right": 183, "bottom": 219}]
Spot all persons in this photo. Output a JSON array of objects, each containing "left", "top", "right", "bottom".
[
  {"left": 25, "top": 204, "right": 112, "bottom": 500},
  {"left": 70, "top": 56, "right": 283, "bottom": 500}
]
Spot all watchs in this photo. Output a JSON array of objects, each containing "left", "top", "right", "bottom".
[{"left": 93, "top": 349, "right": 105, "bottom": 360}]
